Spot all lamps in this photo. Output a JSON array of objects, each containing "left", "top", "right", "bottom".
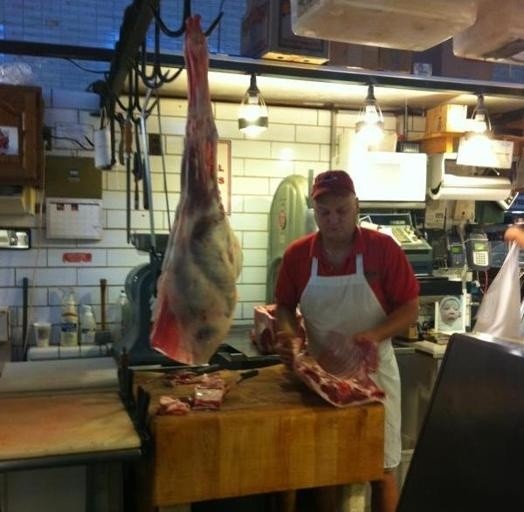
[
  {"left": 238, "top": 73, "right": 269, "bottom": 138},
  {"left": 355, "top": 85, "right": 386, "bottom": 147},
  {"left": 456, "top": 94, "right": 499, "bottom": 168}
]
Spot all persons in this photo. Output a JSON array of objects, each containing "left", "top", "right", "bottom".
[
  {"left": 504, "top": 227, "right": 524, "bottom": 249},
  {"left": 439, "top": 295, "right": 462, "bottom": 327},
  {"left": 274, "top": 171, "right": 420, "bottom": 512}
]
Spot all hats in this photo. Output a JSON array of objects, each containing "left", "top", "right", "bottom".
[{"left": 311, "top": 170, "right": 356, "bottom": 200}]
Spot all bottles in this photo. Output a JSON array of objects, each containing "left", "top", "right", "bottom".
[{"left": 60, "top": 300, "right": 96, "bottom": 345}]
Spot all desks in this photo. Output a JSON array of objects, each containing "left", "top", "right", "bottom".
[
  {"left": 0, "top": 391, "right": 141, "bottom": 512},
  {"left": 137, "top": 364, "right": 385, "bottom": 512}
]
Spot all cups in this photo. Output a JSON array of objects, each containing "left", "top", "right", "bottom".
[{"left": 34, "top": 323, "right": 53, "bottom": 346}]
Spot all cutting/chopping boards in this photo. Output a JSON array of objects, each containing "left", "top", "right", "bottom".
[{"left": 1, "top": 391, "right": 143, "bottom": 460}]
